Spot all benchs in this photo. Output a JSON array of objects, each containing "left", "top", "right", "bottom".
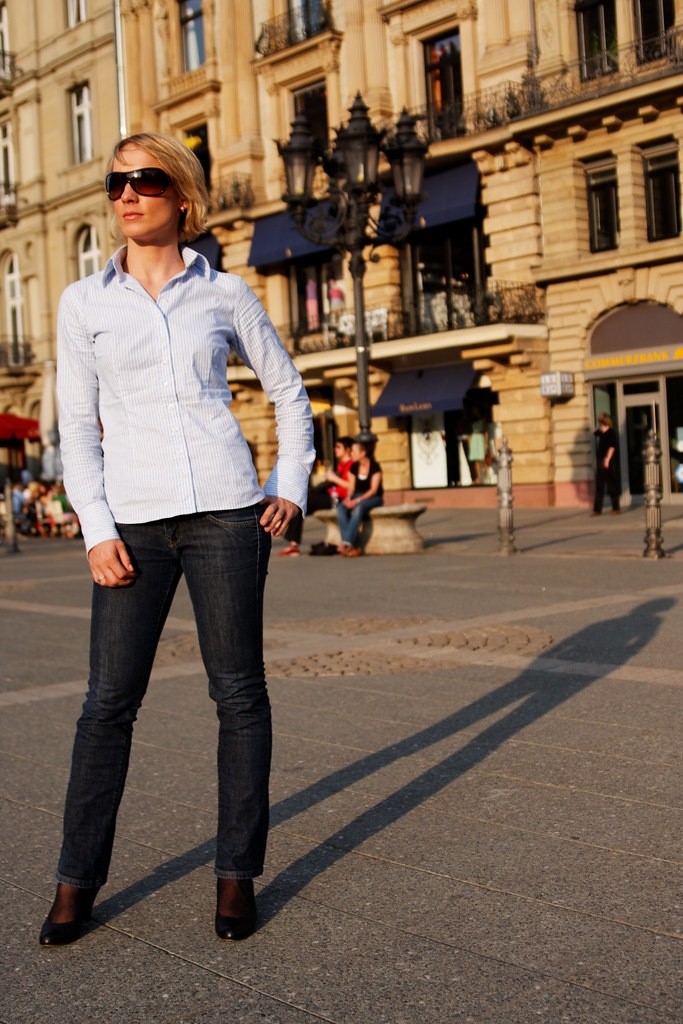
[{"left": 314, "top": 504, "right": 427, "bottom": 555}]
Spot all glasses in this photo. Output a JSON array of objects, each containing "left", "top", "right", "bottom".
[
  {"left": 104, "top": 166, "right": 176, "bottom": 201},
  {"left": 336, "top": 445, "right": 343, "bottom": 450}
]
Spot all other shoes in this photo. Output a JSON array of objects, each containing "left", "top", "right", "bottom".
[
  {"left": 321, "top": 544, "right": 338, "bottom": 555},
  {"left": 276, "top": 545, "right": 301, "bottom": 558},
  {"left": 610, "top": 509, "right": 621, "bottom": 515},
  {"left": 337, "top": 542, "right": 364, "bottom": 557},
  {"left": 589, "top": 510, "right": 603, "bottom": 518},
  {"left": 308, "top": 541, "right": 327, "bottom": 556}
]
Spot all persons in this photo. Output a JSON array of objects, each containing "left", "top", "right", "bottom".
[
  {"left": 273, "top": 429, "right": 384, "bottom": 560},
  {"left": 0, "top": 456, "right": 82, "bottom": 556},
  {"left": 38, "top": 132, "right": 317, "bottom": 949},
  {"left": 587, "top": 412, "right": 623, "bottom": 517}
]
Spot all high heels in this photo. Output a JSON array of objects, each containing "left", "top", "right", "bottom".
[
  {"left": 39, "top": 885, "right": 101, "bottom": 947},
  {"left": 215, "top": 878, "right": 259, "bottom": 941}
]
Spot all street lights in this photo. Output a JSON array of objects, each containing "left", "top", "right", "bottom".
[{"left": 267, "top": 92, "right": 430, "bottom": 445}]
